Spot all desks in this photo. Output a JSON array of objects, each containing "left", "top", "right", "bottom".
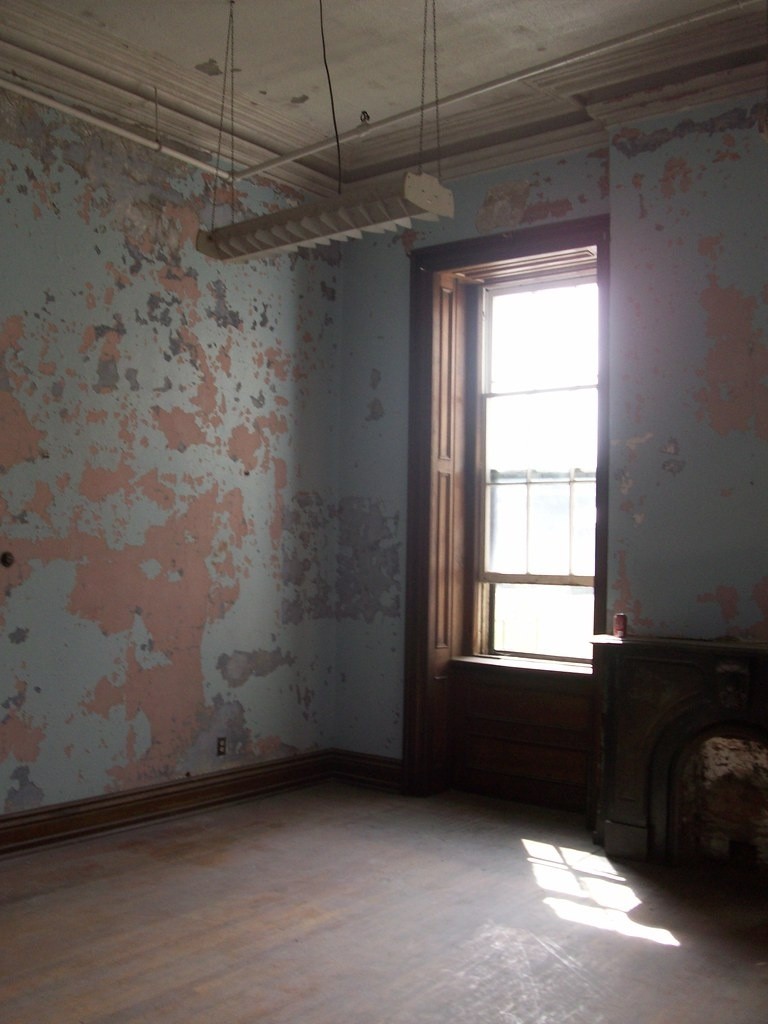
[{"left": 587, "top": 634, "right": 767, "bottom": 877}]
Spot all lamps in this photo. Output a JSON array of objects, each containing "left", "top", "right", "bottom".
[{"left": 196, "top": 0, "right": 455, "bottom": 262}]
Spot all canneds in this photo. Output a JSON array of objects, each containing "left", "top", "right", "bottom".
[{"left": 613, "top": 613, "right": 627, "bottom": 637}]
